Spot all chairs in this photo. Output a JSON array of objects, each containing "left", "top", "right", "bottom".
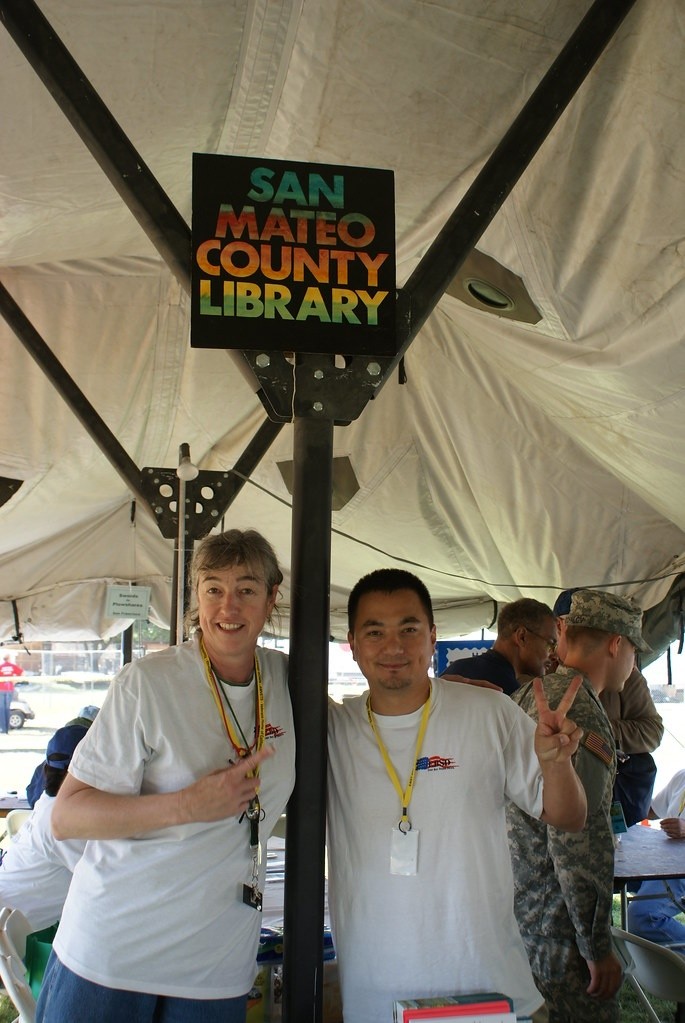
[
  {"left": 611, "top": 925, "right": 685, "bottom": 1023},
  {"left": 0, "top": 809, "right": 34, "bottom": 853},
  {"left": 0, "top": 905, "right": 53, "bottom": 1023}
]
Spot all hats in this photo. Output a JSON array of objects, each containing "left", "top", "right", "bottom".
[
  {"left": 45, "top": 724, "right": 89, "bottom": 769},
  {"left": 552, "top": 588, "right": 586, "bottom": 616},
  {"left": 66, "top": 717, "right": 92, "bottom": 729},
  {"left": 79, "top": 705, "right": 100, "bottom": 721},
  {"left": 564, "top": 589, "right": 654, "bottom": 654}
]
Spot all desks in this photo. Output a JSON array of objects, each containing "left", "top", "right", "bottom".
[
  {"left": 255, "top": 835, "right": 335, "bottom": 967},
  {"left": 613, "top": 825, "right": 685, "bottom": 934},
  {"left": 0, "top": 798, "right": 32, "bottom": 819}
]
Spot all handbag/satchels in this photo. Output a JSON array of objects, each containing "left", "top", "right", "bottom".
[{"left": 612, "top": 749, "right": 658, "bottom": 827}]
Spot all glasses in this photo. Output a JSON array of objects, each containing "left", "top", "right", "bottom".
[{"left": 512, "top": 624, "right": 558, "bottom": 652}]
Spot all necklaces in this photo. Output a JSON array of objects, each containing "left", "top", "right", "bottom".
[{"left": 212, "top": 662, "right": 263, "bottom": 913}]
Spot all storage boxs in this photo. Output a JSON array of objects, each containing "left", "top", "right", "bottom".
[{"left": 393, "top": 992, "right": 514, "bottom": 1023}]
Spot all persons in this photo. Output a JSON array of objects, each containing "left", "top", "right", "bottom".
[
  {"left": 627, "top": 770, "right": 685, "bottom": 957},
  {"left": 327, "top": 569, "right": 586, "bottom": 1023},
  {"left": 442, "top": 599, "right": 559, "bottom": 696},
  {"left": 554, "top": 588, "right": 663, "bottom": 755},
  {"left": 0, "top": 706, "right": 99, "bottom": 932},
  {"left": 0, "top": 655, "right": 25, "bottom": 734},
  {"left": 34, "top": 529, "right": 503, "bottom": 1022},
  {"left": 504, "top": 590, "right": 653, "bottom": 1023}
]
